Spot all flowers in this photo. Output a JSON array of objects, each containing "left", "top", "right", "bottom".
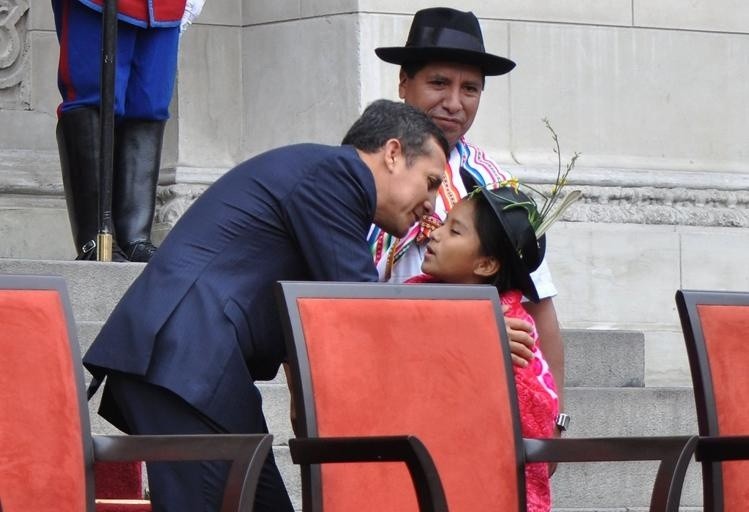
[{"left": 469, "top": 118, "right": 586, "bottom": 258}]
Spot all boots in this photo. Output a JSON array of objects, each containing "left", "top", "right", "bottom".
[
  {"left": 56, "top": 108, "right": 125, "bottom": 261},
  {"left": 114, "top": 119, "right": 162, "bottom": 260}
]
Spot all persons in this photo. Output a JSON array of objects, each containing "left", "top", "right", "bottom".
[
  {"left": 79, "top": 96, "right": 537, "bottom": 512},
  {"left": 404, "top": 186, "right": 560, "bottom": 512},
  {"left": 365, "top": 5, "right": 563, "bottom": 482},
  {"left": 50, "top": 1, "right": 206, "bottom": 262}
]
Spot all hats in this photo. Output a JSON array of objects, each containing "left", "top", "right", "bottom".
[
  {"left": 459, "top": 163, "right": 550, "bottom": 305},
  {"left": 376, "top": 6, "right": 516, "bottom": 77}
]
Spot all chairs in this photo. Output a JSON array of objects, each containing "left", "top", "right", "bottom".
[
  {"left": 274, "top": 280, "right": 698, "bottom": 512},
  {"left": 0, "top": 276, "right": 273, "bottom": 512},
  {"left": 676, "top": 288, "right": 749, "bottom": 512}
]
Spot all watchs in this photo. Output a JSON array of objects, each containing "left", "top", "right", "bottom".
[{"left": 555, "top": 412, "right": 571, "bottom": 432}]
